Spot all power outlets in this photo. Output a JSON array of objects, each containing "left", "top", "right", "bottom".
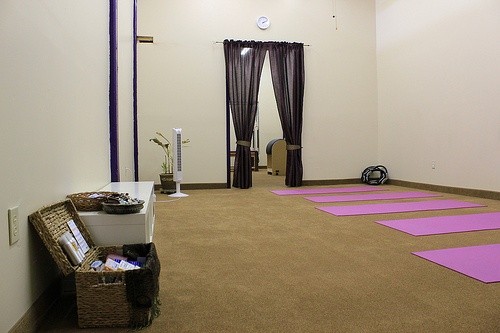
[
  {"left": 431, "top": 161, "right": 435, "bottom": 169},
  {"left": 9, "top": 206, "right": 20, "bottom": 246}
]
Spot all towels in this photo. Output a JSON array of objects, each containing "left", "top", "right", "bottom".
[{"left": 123, "top": 242, "right": 162, "bottom": 329}]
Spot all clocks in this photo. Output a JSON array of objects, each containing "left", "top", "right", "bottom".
[{"left": 257, "top": 16, "right": 271, "bottom": 30}]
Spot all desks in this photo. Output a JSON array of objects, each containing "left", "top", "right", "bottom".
[
  {"left": 73, "top": 182, "right": 157, "bottom": 243},
  {"left": 230, "top": 150, "right": 259, "bottom": 171}
]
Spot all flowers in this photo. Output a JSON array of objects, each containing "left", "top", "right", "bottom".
[{"left": 150, "top": 132, "right": 190, "bottom": 173}]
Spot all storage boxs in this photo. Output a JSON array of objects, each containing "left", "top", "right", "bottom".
[{"left": 27, "top": 198, "right": 162, "bottom": 330}]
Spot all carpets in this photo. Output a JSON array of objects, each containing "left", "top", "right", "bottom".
[
  {"left": 270, "top": 186, "right": 388, "bottom": 196},
  {"left": 313, "top": 199, "right": 489, "bottom": 216},
  {"left": 373, "top": 212, "right": 499, "bottom": 236},
  {"left": 302, "top": 191, "right": 445, "bottom": 203},
  {"left": 410, "top": 243, "right": 499, "bottom": 284}
]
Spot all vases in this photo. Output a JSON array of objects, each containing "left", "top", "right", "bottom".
[{"left": 159, "top": 174, "right": 179, "bottom": 193}]
